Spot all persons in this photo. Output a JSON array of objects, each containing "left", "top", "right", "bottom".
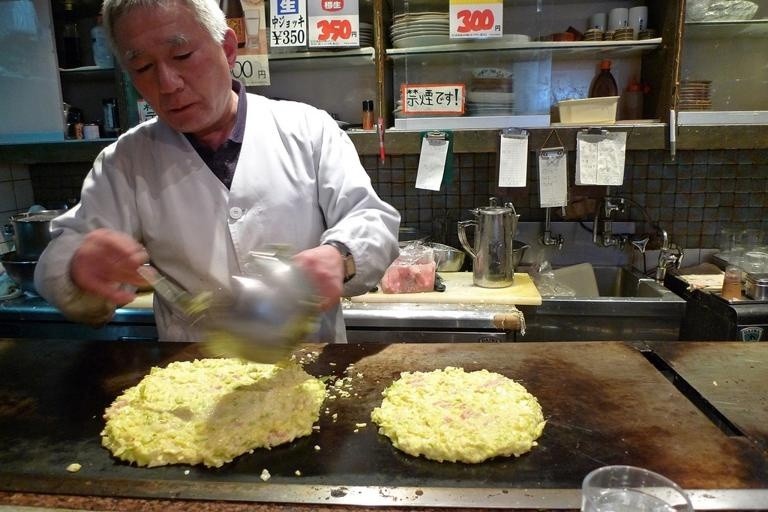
[{"left": 34, "top": 0, "right": 403, "bottom": 343}]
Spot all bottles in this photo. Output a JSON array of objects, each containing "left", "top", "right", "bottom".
[
  {"left": 361, "top": 99, "right": 376, "bottom": 131},
  {"left": 67, "top": 107, "right": 85, "bottom": 140},
  {"left": 219, "top": 0, "right": 247, "bottom": 48},
  {"left": 101, "top": 96, "right": 121, "bottom": 129},
  {"left": 431, "top": 215, "right": 455, "bottom": 246},
  {"left": 622, "top": 79, "right": 645, "bottom": 121},
  {"left": 57, "top": 0, "right": 115, "bottom": 70},
  {"left": 589, "top": 58, "right": 619, "bottom": 98}
]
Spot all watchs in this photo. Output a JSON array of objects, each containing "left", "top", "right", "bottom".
[{"left": 324, "top": 240, "right": 356, "bottom": 283}]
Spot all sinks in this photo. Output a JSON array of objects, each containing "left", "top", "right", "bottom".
[{"left": 516, "top": 263, "right": 688, "bottom": 304}]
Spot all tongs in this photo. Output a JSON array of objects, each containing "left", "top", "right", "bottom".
[{"left": 137, "top": 260, "right": 192, "bottom": 318}]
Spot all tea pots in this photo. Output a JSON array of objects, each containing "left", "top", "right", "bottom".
[{"left": 456, "top": 196, "right": 522, "bottom": 290}]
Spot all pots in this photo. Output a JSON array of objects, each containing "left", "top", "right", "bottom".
[{"left": 9, "top": 208, "right": 70, "bottom": 261}]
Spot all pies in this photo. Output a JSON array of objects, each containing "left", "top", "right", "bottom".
[
  {"left": 101, "top": 356, "right": 326, "bottom": 468},
  {"left": 371, "top": 366, "right": 548, "bottom": 464}
]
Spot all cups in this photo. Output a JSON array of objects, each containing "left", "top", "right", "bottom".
[
  {"left": 743, "top": 251, "right": 768, "bottom": 275},
  {"left": 589, "top": 5, "right": 648, "bottom": 40},
  {"left": 578, "top": 464, "right": 694, "bottom": 512},
  {"left": 244, "top": 8, "right": 261, "bottom": 47},
  {"left": 721, "top": 264, "right": 743, "bottom": 301}
]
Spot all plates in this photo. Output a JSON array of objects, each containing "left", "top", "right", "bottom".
[
  {"left": 466, "top": 66, "right": 520, "bottom": 115},
  {"left": 474, "top": 32, "right": 530, "bottom": 43},
  {"left": 358, "top": 21, "right": 375, "bottom": 47},
  {"left": 389, "top": 11, "right": 467, "bottom": 49},
  {"left": 677, "top": 79, "right": 714, "bottom": 111}
]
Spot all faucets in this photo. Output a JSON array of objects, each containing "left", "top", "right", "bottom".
[
  {"left": 542, "top": 204, "right": 567, "bottom": 251},
  {"left": 650, "top": 222, "right": 679, "bottom": 285}
]
